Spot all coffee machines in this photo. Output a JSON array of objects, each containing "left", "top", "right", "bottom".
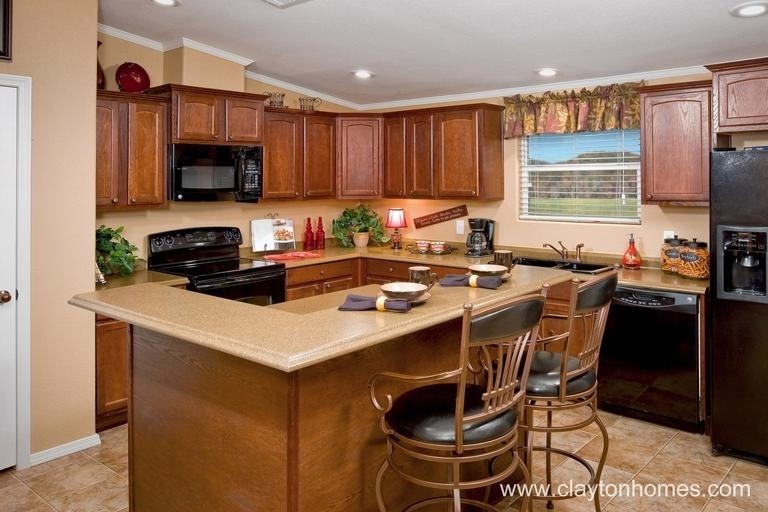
[{"left": 463, "top": 218, "right": 497, "bottom": 259}]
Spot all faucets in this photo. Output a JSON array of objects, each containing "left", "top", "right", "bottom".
[
  {"left": 543, "top": 240, "right": 569, "bottom": 259},
  {"left": 576, "top": 243, "right": 585, "bottom": 264}
]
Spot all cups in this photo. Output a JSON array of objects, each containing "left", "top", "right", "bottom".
[
  {"left": 299, "top": 97, "right": 323, "bottom": 111},
  {"left": 407, "top": 265, "right": 439, "bottom": 290},
  {"left": 262, "top": 91, "right": 286, "bottom": 106},
  {"left": 494, "top": 248, "right": 519, "bottom": 271}
]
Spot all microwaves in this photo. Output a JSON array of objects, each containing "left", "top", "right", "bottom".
[{"left": 167, "top": 143, "right": 263, "bottom": 201}]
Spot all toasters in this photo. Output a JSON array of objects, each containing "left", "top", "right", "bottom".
[{"left": 147, "top": 227, "right": 286, "bottom": 286}]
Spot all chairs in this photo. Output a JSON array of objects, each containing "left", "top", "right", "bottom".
[
  {"left": 484, "top": 266, "right": 620, "bottom": 512},
  {"left": 366, "top": 279, "right": 551, "bottom": 512}
]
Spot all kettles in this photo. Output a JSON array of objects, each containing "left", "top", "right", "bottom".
[{"left": 732, "top": 251, "right": 764, "bottom": 289}]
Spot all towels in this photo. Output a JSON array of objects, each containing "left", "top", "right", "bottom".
[
  {"left": 439, "top": 272, "right": 504, "bottom": 290},
  {"left": 338, "top": 293, "right": 412, "bottom": 314}
]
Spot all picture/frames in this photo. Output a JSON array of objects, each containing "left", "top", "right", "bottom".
[{"left": 0, "top": 0, "right": 13, "bottom": 62}]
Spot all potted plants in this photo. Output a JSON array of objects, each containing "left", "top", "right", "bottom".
[
  {"left": 332, "top": 202, "right": 388, "bottom": 250},
  {"left": 94, "top": 221, "right": 139, "bottom": 284}
]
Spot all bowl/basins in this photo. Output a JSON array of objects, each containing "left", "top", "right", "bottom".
[
  {"left": 430, "top": 240, "right": 448, "bottom": 254},
  {"left": 467, "top": 264, "right": 508, "bottom": 276},
  {"left": 381, "top": 282, "right": 429, "bottom": 300},
  {"left": 415, "top": 240, "right": 431, "bottom": 253}
]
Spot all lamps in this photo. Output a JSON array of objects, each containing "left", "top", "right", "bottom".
[{"left": 387, "top": 206, "right": 408, "bottom": 250}]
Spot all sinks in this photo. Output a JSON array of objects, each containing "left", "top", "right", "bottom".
[
  {"left": 557, "top": 262, "right": 614, "bottom": 274},
  {"left": 511, "top": 256, "right": 561, "bottom": 268}
]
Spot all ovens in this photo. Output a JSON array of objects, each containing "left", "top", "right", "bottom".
[{"left": 597, "top": 285, "right": 711, "bottom": 434}]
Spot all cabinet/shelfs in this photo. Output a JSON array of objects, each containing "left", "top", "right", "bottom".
[
  {"left": 336, "top": 111, "right": 382, "bottom": 202},
  {"left": 261, "top": 105, "right": 336, "bottom": 202},
  {"left": 96, "top": 313, "right": 131, "bottom": 433},
  {"left": 170, "top": 90, "right": 266, "bottom": 144},
  {"left": 94, "top": 93, "right": 168, "bottom": 208},
  {"left": 712, "top": 61, "right": 766, "bottom": 134},
  {"left": 384, "top": 111, "right": 432, "bottom": 199},
  {"left": 435, "top": 102, "right": 503, "bottom": 201},
  {"left": 286, "top": 259, "right": 361, "bottom": 302},
  {"left": 364, "top": 259, "right": 470, "bottom": 285},
  {"left": 540, "top": 280, "right": 595, "bottom": 358},
  {"left": 638, "top": 79, "right": 711, "bottom": 207}
]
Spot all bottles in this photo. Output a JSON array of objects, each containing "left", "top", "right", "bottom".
[
  {"left": 622, "top": 234, "right": 640, "bottom": 269},
  {"left": 661, "top": 234, "right": 688, "bottom": 274},
  {"left": 677, "top": 238, "right": 710, "bottom": 280},
  {"left": 316, "top": 217, "right": 325, "bottom": 250},
  {"left": 305, "top": 218, "right": 314, "bottom": 251}
]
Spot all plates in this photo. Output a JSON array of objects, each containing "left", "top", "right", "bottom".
[
  {"left": 114, "top": 62, "right": 150, "bottom": 92},
  {"left": 465, "top": 272, "right": 513, "bottom": 282},
  {"left": 377, "top": 291, "right": 432, "bottom": 306}
]
